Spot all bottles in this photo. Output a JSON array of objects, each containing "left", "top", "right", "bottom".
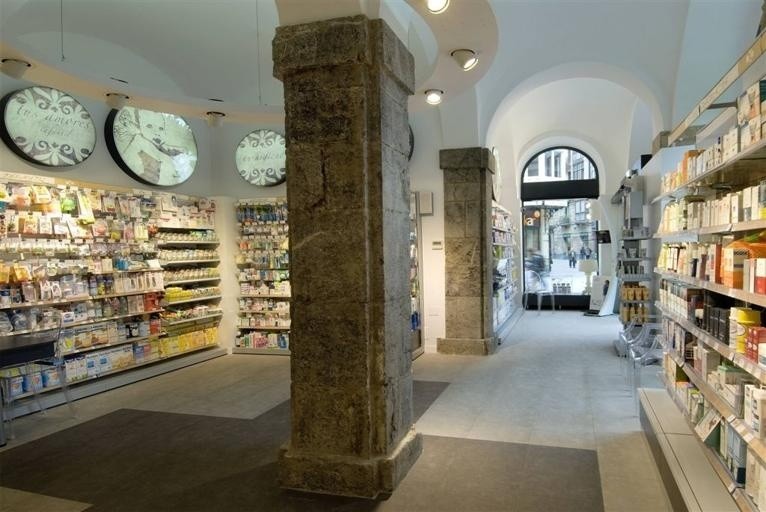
[
  {"left": 552, "top": 283, "right": 570, "bottom": 295},
  {"left": 619, "top": 284, "right": 647, "bottom": 324}
]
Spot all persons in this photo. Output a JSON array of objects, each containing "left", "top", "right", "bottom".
[
  {"left": 580, "top": 247, "right": 585, "bottom": 260},
  {"left": 568, "top": 248, "right": 576, "bottom": 268},
  {"left": 586, "top": 247, "right": 592, "bottom": 260},
  {"left": 123, "top": 110, "right": 187, "bottom": 185}
]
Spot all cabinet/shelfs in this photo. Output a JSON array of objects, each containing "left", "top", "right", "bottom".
[
  {"left": 641, "top": 29, "right": 765, "bottom": 512},
  {"left": 145, "top": 195, "right": 225, "bottom": 363},
  {"left": 0, "top": 170, "right": 166, "bottom": 424},
  {"left": 489, "top": 202, "right": 518, "bottom": 332},
  {"left": 231, "top": 197, "right": 422, "bottom": 363},
  {"left": 607, "top": 167, "right": 650, "bottom": 355}
]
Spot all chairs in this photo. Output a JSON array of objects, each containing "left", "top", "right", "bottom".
[{"left": 524, "top": 269, "right": 555, "bottom": 314}]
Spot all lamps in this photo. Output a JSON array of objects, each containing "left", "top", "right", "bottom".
[
  {"left": 576, "top": 259, "right": 598, "bottom": 295},
  {"left": 450, "top": 48, "right": 479, "bottom": 71},
  {"left": 103, "top": 91, "right": 129, "bottom": 109},
  {"left": 205, "top": 111, "right": 225, "bottom": 128},
  {"left": 424, "top": 89, "right": 444, "bottom": 105},
  {"left": 0, "top": 57, "right": 31, "bottom": 80}
]
